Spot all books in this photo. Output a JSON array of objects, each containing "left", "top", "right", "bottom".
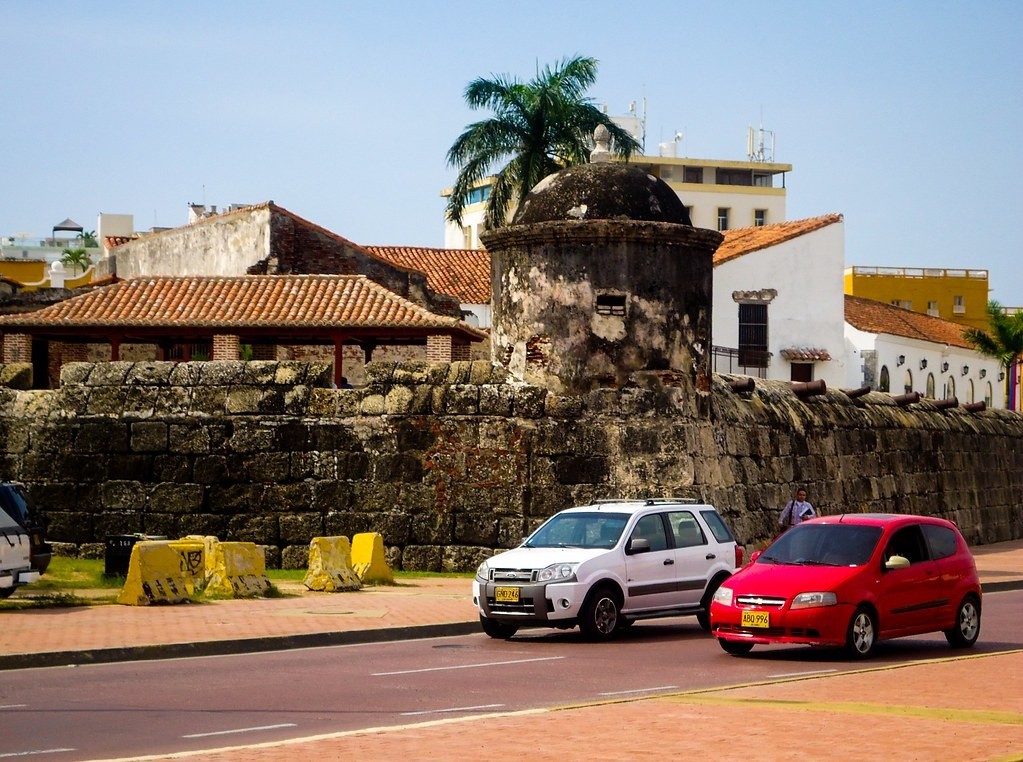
[{"left": 799, "top": 508, "right": 812, "bottom": 518}]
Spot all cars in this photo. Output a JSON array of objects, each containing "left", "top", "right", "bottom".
[{"left": 709, "top": 513, "right": 982, "bottom": 660}]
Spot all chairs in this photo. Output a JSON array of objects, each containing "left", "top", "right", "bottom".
[
  {"left": 592, "top": 522, "right": 621, "bottom": 545},
  {"left": 636, "top": 521, "right": 661, "bottom": 549},
  {"left": 677, "top": 521, "right": 698, "bottom": 547}
]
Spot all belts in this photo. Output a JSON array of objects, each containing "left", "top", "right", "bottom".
[{"left": 790, "top": 523, "right": 795, "bottom": 526}]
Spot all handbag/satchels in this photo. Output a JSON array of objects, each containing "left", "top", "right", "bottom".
[{"left": 779, "top": 526, "right": 788, "bottom": 532}]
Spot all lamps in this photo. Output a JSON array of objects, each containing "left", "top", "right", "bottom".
[
  {"left": 1017, "top": 376, "right": 1020, "bottom": 384},
  {"left": 921, "top": 358, "right": 927, "bottom": 370},
  {"left": 897, "top": 355, "right": 905, "bottom": 367},
  {"left": 998, "top": 372, "right": 1005, "bottom": 381},
  {"left": 962, "top": 365, "right": 969, "bottom": 377},
  {"left": 980, "top": 368, "right": 986, "bottom": 379},
  {"left": 941, "top": 362, "right": 949, "bottom": 373}
]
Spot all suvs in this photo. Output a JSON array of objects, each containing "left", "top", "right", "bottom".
[{"left": 472, "top": 498, "right": 743, "bottom": 643}]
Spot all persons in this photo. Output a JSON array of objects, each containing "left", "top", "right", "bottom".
[
  {"left": 630, "top": 526, "right": 641, "bottom": 549},
  {"left": 862, "top": 532, "right": 904, "bottom": 564},
  {"left": 778, "top": 489, "right": 816, "bottom": 529}
]
